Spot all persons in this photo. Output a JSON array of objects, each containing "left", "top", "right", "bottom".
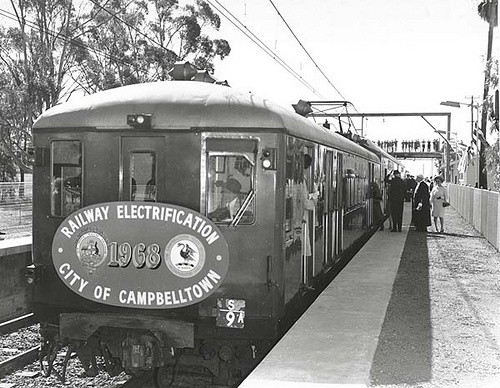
[
  {"left": 370, "top": 183, "right": 386, "bottom": 230},
  {"left": 64, "top": 157, "right": 82, "bottom": 188},
  {"left": 206, "top": 178, "right": 254, "bottom": 225},
  {"left": 428, "top": 175, "right": 448, "bottom": 233},
  {"left": 300, "top": 154, "right": 319, "bottom": 258},
  {"left": 389, "top": 169, "right": 407, "bottom": 233},
  {"left": 404, "top": 173, "right": 417, "bottom": 203},
  {"left": 412, "top": 175, "right": 432, "bottom": 231}
]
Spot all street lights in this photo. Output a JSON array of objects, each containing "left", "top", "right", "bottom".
[{"left": 440, "top": 101, "right": 478, "bottom": 188}]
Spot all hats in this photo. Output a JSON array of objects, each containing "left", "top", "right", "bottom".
[
  {"left": 417, "top": 174, "right": 425, "bottom": 179},
  {"left": 393, "top": 170, "right": 400, "bottom": 175},
  {"left": 221, "top": 180, "right": 246, "bottom": 197},
  {"left": 435, "top": 176, "right": 445, "bottom": 182}
]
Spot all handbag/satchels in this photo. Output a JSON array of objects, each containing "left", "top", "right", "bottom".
[{"left": 443, "top": 200, "right": 451, "bottom": 207}]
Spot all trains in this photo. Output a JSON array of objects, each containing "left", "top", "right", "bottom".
[{"left": 21, "top": 61, "right": 409, "bottom": 388}]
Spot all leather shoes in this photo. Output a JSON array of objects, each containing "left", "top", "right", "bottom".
[{"left": 391, "top": 230, "right": 397, "bottom": 232}]
